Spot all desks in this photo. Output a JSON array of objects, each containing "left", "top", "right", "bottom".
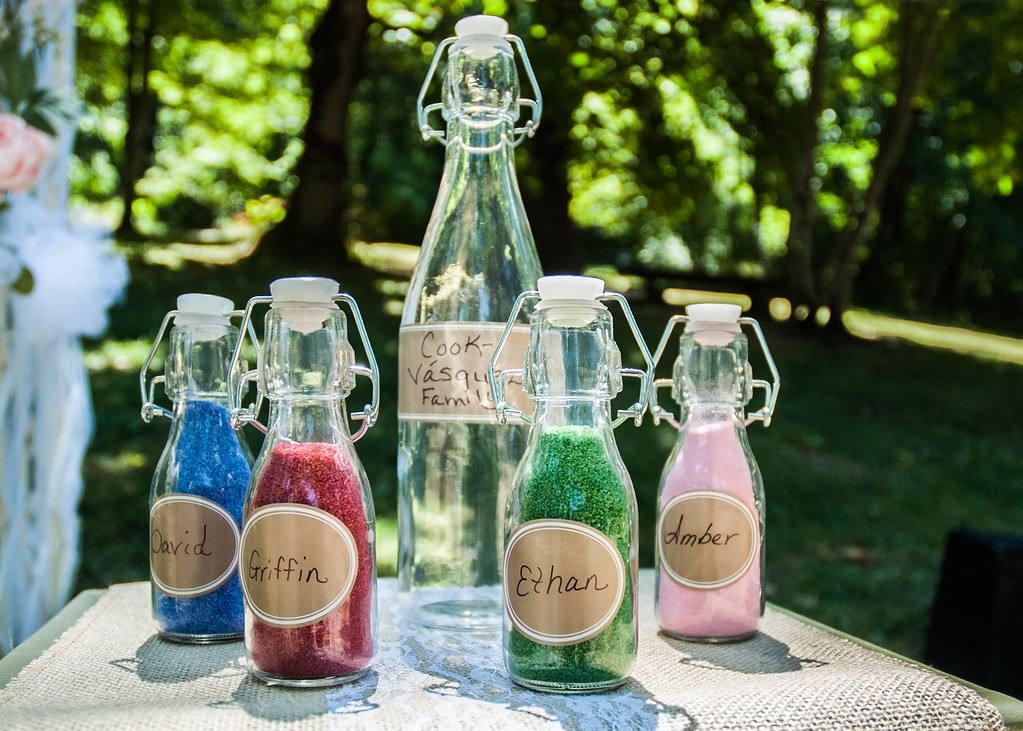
[{"left": 0, "top": 589, "right": 1023, "bottom": 731}]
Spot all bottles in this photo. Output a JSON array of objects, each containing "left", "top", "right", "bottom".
[
  {"left": 487, "top": 274, "right": 651, "bottom": 693},
  {"left": 226, "top": 275, "right": 379, "bottom": 688},
  {"left": 139, "top": 289, "right": 262, "bottom": 643},
  {"left": 397, "top": 13, "right": 550, "bottom": 661},
  {"left": 648, "top": 304, "right": 781, "bottom": 645}
]
